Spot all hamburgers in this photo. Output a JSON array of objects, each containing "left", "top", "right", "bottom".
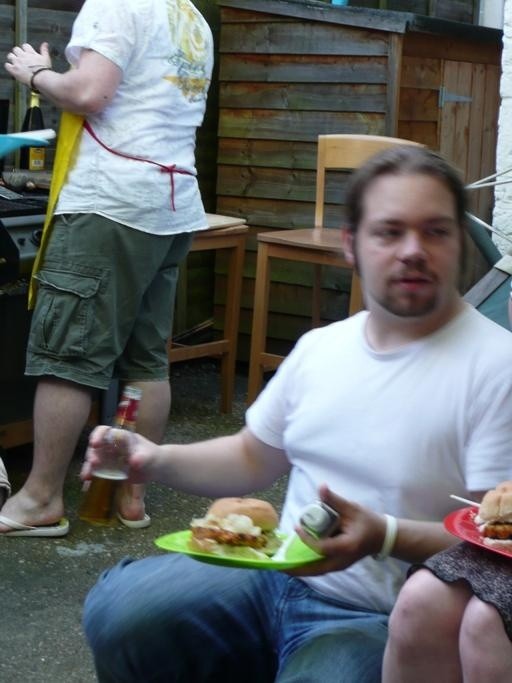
[
  {"left": 475, "top": 481, "right": 512, "bottom": 547},
  {"left": 187, "top": 498, "right": 283, "bottom": 556}
]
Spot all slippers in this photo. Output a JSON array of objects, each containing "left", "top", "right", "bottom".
[
  {"left": 0, "top": 513, "right": 69, "bottom": 540},
  {"left": 116, "top": 511, "right": 152, "bottom": 529}
]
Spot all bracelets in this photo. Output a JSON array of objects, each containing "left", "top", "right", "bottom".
[{"left": 29, "top": 67, "right": 57, "bottom": 95}]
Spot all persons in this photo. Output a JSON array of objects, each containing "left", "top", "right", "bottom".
[{"left": 0, "top": 1, "right": 213, "bottom": 536}]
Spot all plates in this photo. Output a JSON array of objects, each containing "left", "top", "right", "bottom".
[
  {"left": 442, "top": 505, "right": 512, "bottom": 561},
  {"left": 153, "top": 528, "right": 327, "bottom": 573}
]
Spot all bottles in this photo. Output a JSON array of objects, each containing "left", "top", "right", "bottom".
[
  {"left": 298, "top": 500, "right": 341, "bottom": 542},
  {"left": 77, "top": 382, "right": 145, "bottom": 528},
  {"left": 18, "top": 87, "right": 47, "bottom": 172}
]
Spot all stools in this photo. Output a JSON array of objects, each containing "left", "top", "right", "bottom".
[
  {"left": 248, "top": 134, "right": 428, "bottom": 406},
  {"left": 168, "top": 212, "right": 250, "bottom": 412}
]
